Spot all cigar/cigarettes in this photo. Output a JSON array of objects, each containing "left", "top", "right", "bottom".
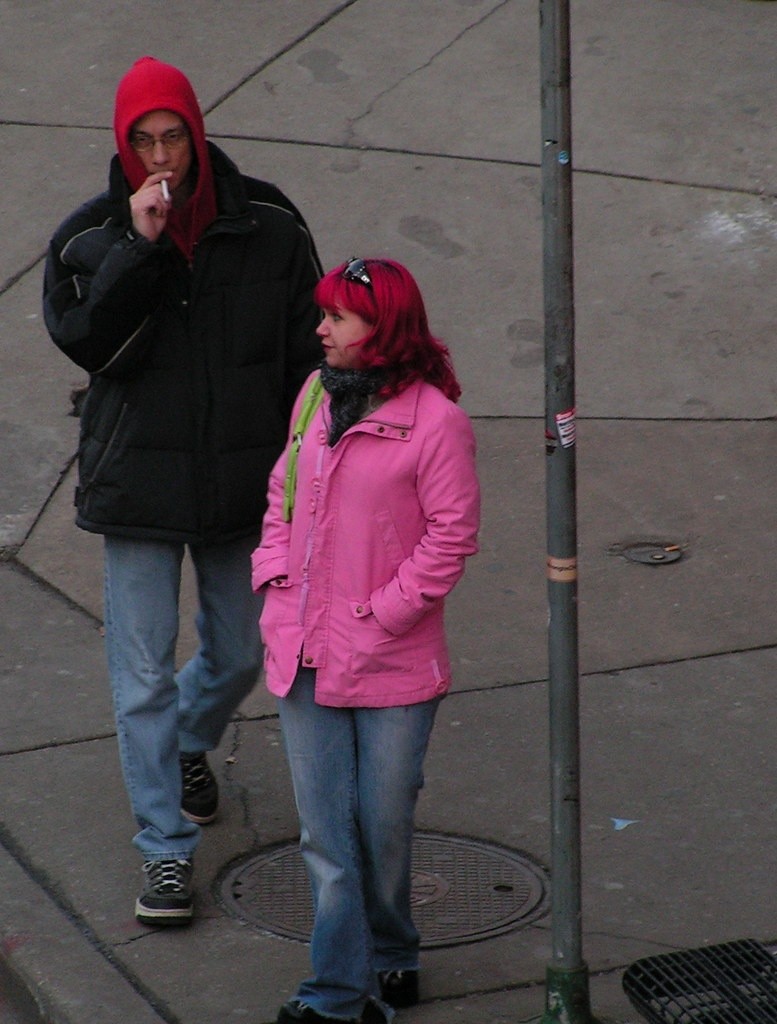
[{"left": 161, "top": 178, "right": 170, "bottom": 203}]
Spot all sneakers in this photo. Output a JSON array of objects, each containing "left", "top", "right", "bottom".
[
  {"left": 278, "top": 1000, "right": 387, "bottom": 1024},
  {"left": 378, "top": 971, "right": 418, "bottom": 1008},
  {"left": 135, "top": 857, "right": 194, "bottom": 919},
  {"left": 180, "top": 751, "right": 219, "bottom": 823}
]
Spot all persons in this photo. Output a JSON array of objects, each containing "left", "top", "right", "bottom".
[
  {"left": 43, "top": 57, "right": 328, "bottom": 927},
  {"left": 252, "top": 256, "right": 480, "bottom": 1024}
]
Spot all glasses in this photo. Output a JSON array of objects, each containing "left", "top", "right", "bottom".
[
  {"left": 127, "top": 129, "right": 188, "bottom": 151},
  {"left": 339, "top": 258, "right": 372, "bottom": 291}
]
